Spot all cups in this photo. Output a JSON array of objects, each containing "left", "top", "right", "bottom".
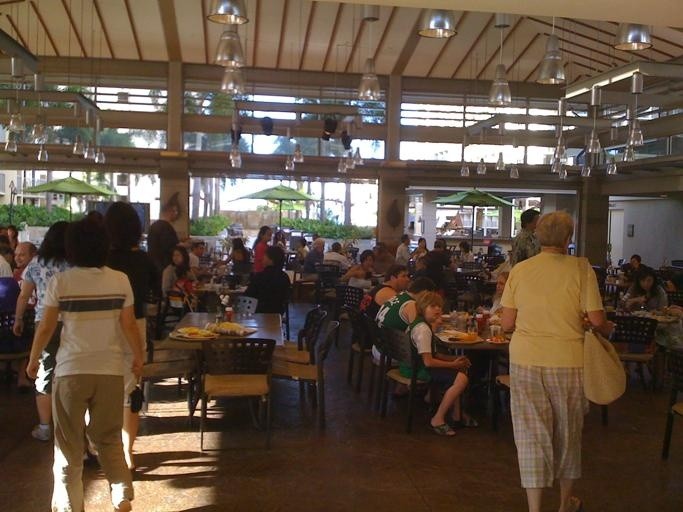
[
  {"left": 490, "top": 325, "right": 501, "bottom": 337},
  {"left": 468, "top": 327, "right": 478, "bottom": 335}
]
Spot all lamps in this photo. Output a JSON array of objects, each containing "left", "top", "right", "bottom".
[
  {"left": 414, "top": 9, "right": 658, "bottom": 186},
  {"left": 199, "top": 1, "right": 393, "bottom": 179},
  {"left": 1, "top": 1, "right": 108, "bottom": 169}
]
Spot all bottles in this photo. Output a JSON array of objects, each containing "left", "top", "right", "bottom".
[
  {"left": 225, "top": 306, "right": 233, "bottom": 322},
  {"left": 455, "top": 311, "right": 467, "bottom": 332},
  {"left": 471, "top": 310, "right": 476, "bottom": 327},
  {"left": 482, "top": 311, "right": 490, "bottom": 327},
  {"left": 605, "top": 266, "right": 627, "bottom": 285},
  {"left": 476, "top": 314, "right": 483, "bottom": 333}
]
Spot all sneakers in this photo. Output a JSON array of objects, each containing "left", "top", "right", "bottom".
[{"left": 31, "top": 424, "right": 51, "bottom": 441}]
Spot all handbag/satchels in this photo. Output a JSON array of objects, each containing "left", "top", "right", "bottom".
[
  {"left": 128, "top": 386, "right": 146, "bottom": 412},
  {"left": 583, "top": 330, "right": 627, "bottom": 407}
]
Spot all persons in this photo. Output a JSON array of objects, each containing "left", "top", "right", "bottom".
[
  {"left": 26, "top": 213, "right": 147, "bottom": 512},
  {"left": 343, "top": 242, "right": 478, "bottom": 436},
  {"left": 148, "top": 202, "right": 205, "bottom": 314},
  {"left": 500, "top": 212, "right": 616, "bottom": 511},
  {"left": 293, "top": 233, "right": 351, "bottom": 287},
  {"left": 511, "top": 208, "right": 540, "bottom": 264},
  {"left": 0, "top": 222, "right": 72, "bottom": 437},
  {"left": 397, "top": 235, "right": 504, "bottom": 308},
  {"left": 500, "top": 209, "right": 616, "bottom": 511},
  {"left": 105, "top": 200, "right": 146, "bottom": 480},
  {"left": 622, "top": 254, "right": 683, "bottom": 387},
  {"left": 223, "top": 225, "right": 290, "bottom": 313}
]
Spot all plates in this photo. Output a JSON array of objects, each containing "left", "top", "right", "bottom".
[
  {"left": 440, "top": 314, "right": 453, "bottom": 319},
  {"left": 486, "top": 338, "right": 508, "bottom": 344},
  {"left": 437, "top": 332, "right": 484, "bottom": 346},
  {"left": 614, "top": 304, "right": 682, "bottom": 322},
  {"left": 167, "top": 330, "right": 216, "bottom": 343}
]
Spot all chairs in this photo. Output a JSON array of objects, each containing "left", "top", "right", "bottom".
[
  {"left": 137, "top": 222, "right": 682, "bottom": 460},
  {"left": 1, "top": 276, "right": 37, "bottom": 363}
]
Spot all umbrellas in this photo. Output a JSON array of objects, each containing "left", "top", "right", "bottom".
[
  {"left": 230, "top": 183, "right": 314, "bottom": 228},
  {"left": 431, "top": 185, "right": 515, "bottom": 254},
  {"left": 23, "top": 171, "right": 119, "bottom": 222}
]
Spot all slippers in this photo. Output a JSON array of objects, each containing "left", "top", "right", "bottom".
[
  {"left": 427, "top": 418, "right": 455, "bottom": 436},
  {"left": 452, "top": 414, "right": 478, "bottom": 428}
]
